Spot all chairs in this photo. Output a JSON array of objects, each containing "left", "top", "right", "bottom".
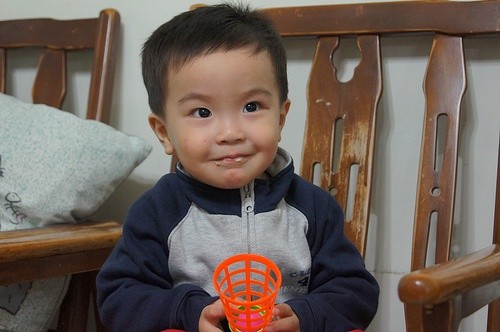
[
  {"left": 0, "top": 6, "right": 133, "bottom": 332},
  {"left": 145, "top": 0, "right": 500, "bottom": 332}
]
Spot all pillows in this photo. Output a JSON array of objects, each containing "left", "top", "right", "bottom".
[{"left": 0, "top": 90, "right": 155, "bottom": 332}]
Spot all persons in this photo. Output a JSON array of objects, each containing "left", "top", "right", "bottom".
[{"left": 94, "top": 0, "right": 380, "bottom": 332}]
